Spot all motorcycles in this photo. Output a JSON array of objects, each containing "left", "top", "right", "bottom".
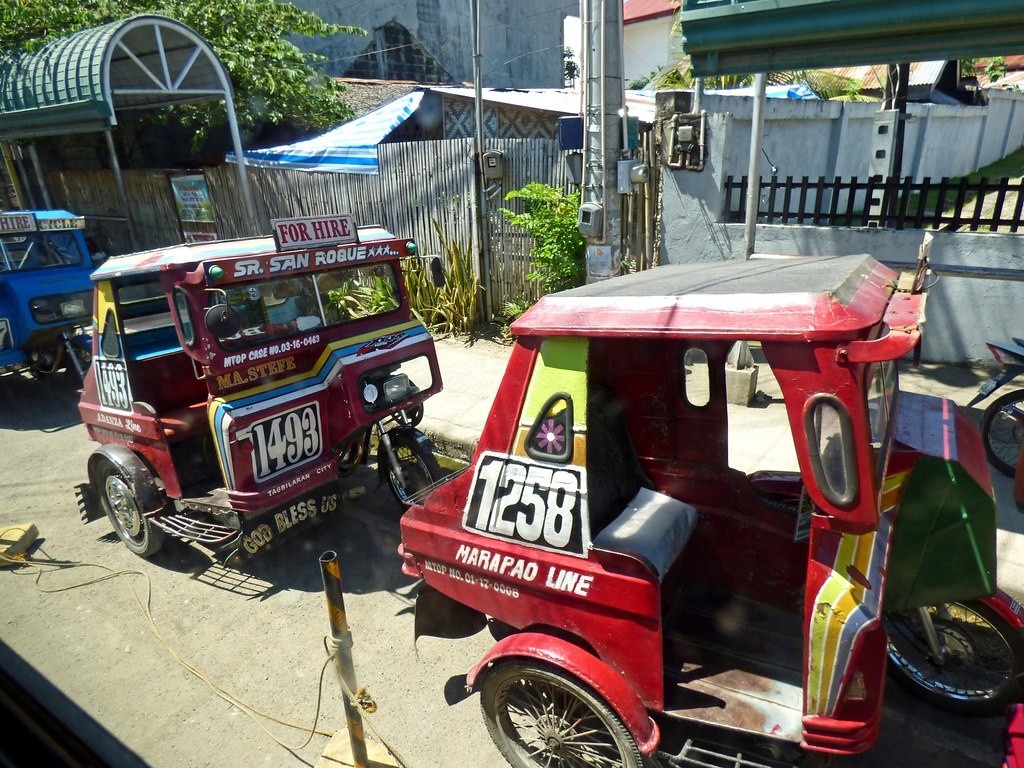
[
  {"left": 76, "top": 212, "right": 446, "bottom": 554},
  {"left": 967, "top": 338, "right": 1022, "bottom": 478},
  {"left": 1, "top": 206, "right": 99, "bottom": 387},
  {"left": 400, "top": 250, "right": 1023, "bottom": 768}
]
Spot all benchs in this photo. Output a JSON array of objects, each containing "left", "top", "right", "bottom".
[
  {"left": 126, "top": 330, "right": 267, "bottom": 488},
  {"left": 586, "top": 382, "right": 704, "bottom": 625}
]
